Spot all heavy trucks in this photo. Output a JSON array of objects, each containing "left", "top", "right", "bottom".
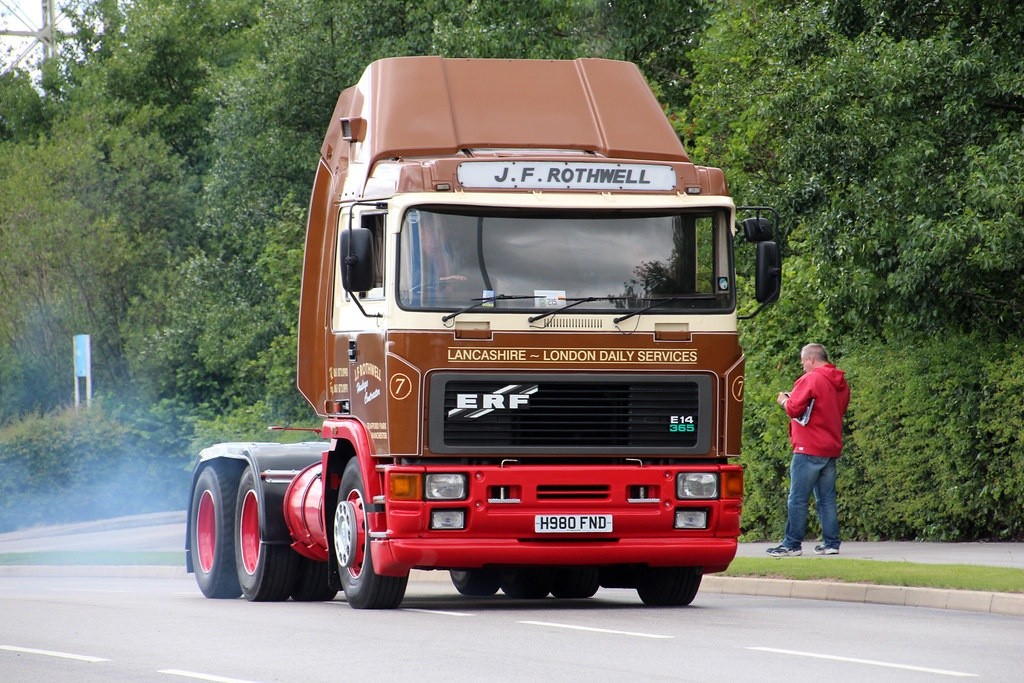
[{"left": 186, "top": 55, "right": 783, "bottom": 612}]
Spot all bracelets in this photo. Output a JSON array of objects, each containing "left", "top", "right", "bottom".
[{"left": 781, "top": 399, "right": 786, "bottom": 409}]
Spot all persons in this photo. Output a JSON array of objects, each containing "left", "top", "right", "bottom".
[{"left": 765, "top": 343, "right": 850, "bottom": 557}]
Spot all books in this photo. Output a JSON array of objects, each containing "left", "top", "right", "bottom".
[{"left": 792, "top": 399, "right": 815, "bottom": 426}]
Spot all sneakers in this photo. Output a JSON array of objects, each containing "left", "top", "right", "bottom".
[
  {"left": 814, "top": 544, "right": 839, "bottom": 554},
  {"left": 766, "top": 545, "right": 803, "bottom": 556}
]
